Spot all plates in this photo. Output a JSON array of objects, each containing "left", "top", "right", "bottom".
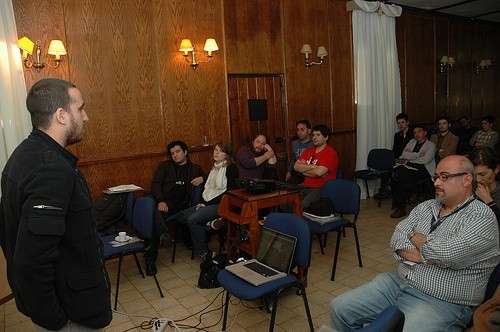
[{"left": 115, "top": 236, "right": 129, "bottom": 242}]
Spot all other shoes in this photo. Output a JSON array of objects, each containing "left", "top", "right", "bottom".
[
  {"left": 373, "top": 193, "right": 388, "bottom": 201},
  {"left": 145, "top": 259, "right": 157, "bottom": 276},
  {"left": 144, "top": 238, "right": 153, "bottom": 250},
  {"left": 201, "top": 249, "right": 217, "bottom": 261},
  {"left": 210, "top": 217, "right": 227, "bottom": 230},
  {"left": 390, "top": 209, "right": 406, "bottom": 218},
  {"left": 184, "top": 238, "right": 194, "bottom": 252}
]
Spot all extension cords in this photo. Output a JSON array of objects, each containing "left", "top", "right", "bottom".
[{"left": 153, "top": 319, "right": 168, "bottom": 332}]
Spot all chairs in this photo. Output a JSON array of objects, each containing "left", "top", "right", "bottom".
[{"left": 101, "top": 140, "right": 500, "bottom": 332}]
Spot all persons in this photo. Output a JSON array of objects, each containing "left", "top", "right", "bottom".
[
  {"left": 374, "top": 112, "right": 500, "bottom": 222},
  {"left": 236, "top": 133, "right": 278, "bottom": 187},
  {"left": 177, "top": 140, "right": 242, "bottom": 263},
  {"left": 0, "top": 77, "right": 112, "bottom": 332},
  {"left": 294, "top": 124, "right": 338, "bottom": 215},
  {"left": 452, "top": 147, "right": 500, "bottom": 301},
  {"left": 327, "top": 154, "right": 500, "bottom": 332},
  {"left": 282, "top": 120, "right": 317, "bottom": 209},
  {"left": 142, "top": 140, "right": 208, "bottom": 276}
]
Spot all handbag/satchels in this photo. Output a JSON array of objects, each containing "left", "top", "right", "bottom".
[
  {"left": 198, "top": 253, "right": 230, "bottom": 289},
  {"left": 303, "top": 191, "right": 335, "bottom": 217},
  {"left": 240, "top": 178, "right": 278, "bottom": 195}
]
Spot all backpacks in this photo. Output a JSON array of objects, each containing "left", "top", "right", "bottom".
[{"left": 95, "top": 192, "right": 127, "bottom": 231}]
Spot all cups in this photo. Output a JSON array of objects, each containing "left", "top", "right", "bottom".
[
  {"left": 119, "top": 232, "right": 126, "bottom": 240},
  {"left": 201, "top": 136, "right": 210, "bottom": 147}
]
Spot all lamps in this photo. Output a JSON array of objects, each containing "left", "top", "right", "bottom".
[
  {"left": 14, "top": 37, "right": 67, "bottom": 73},
  {"left": 301, "top": 44, "right": 328, "bottom": 70},
  {"left": 179, "top": 38, "right": 219, "bottom": 70},
  {"left": 440, "top": 56, "right": 456, "bottom": 73},
  {"left": 476, "top": 59, "right": 492, "bottom": 76}
]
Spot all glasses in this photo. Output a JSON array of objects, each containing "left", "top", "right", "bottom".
[{"left": 430, "top": 172, "right": 467, "bottom": 183}]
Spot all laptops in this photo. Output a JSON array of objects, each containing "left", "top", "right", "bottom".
[{"left": 224, "top": 226, "right": 297, "bottom": 287}]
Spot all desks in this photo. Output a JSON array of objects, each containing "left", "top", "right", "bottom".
[{"left": 218, "top": 188, "right": 307, "bottom": 305}]
